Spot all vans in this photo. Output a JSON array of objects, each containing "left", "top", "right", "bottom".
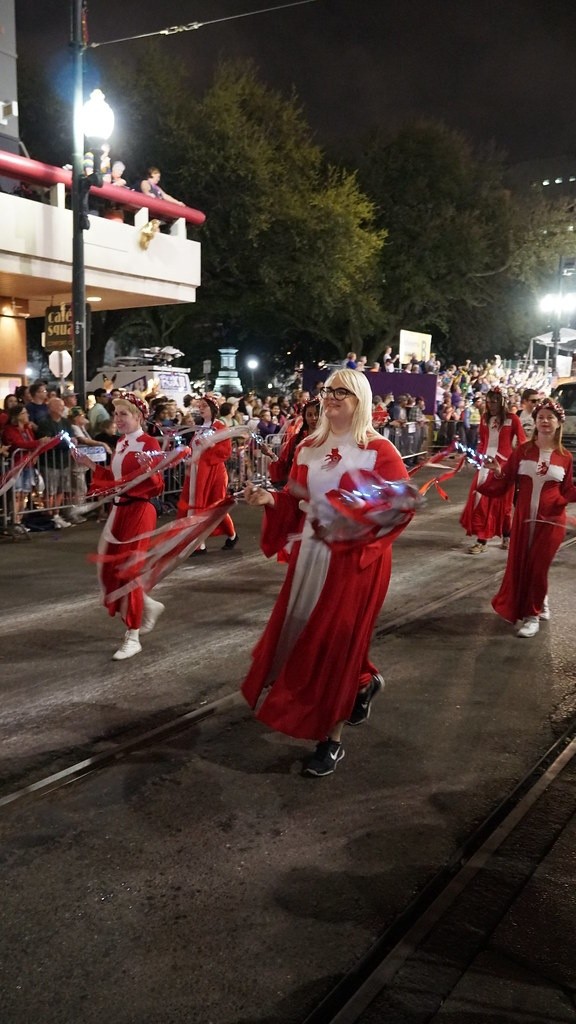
[{"left": 532, "top": 382, "right": 576, "bottom": 484}]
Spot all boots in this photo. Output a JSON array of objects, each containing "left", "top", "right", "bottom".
[
  {"left": 112, "top": 629, "right": 143, "bottom": 660},
  {"left": 138, "top": 594, "right": 164, "bottom": 634}
]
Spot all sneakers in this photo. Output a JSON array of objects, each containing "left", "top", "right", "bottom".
[
  {"left": 5, "top": 508, "right": 113, "bottom": 535},
  {"left": 538, "top": 595, "right": 550, "bottom": 620},
  {"left": 517, "top": 615, "right": 540, "bottom": 637},
  {"left": 346, "top": 672, "right": 385, "bottom": 725},
  {"left": 305, "top": 738, "right": 345, "bottom": 776}
]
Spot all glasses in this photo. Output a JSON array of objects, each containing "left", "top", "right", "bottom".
[
  {"left": 525, "top": 397, "right": 541, "bottom": 403},
  {"left": 34, "top": 389, "right": 46, "bottom": 394},
  {"left": 320, "top": 386, "right": 357, "bottom": 401},
  {"left": 18, "top": 411, "right": 28, "bottom": 416}
]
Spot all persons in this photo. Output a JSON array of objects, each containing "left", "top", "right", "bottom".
[
  {"left": 483, "top": 397, "right": 576, "bottom": 638},
  {"left": 0, "top": 383, "right": 122, "bottom": 531},
  {"left": 72, "top": 392, "right": 165, "bottom": 660},
  {"left": 21, "top": 152, "right": 134, "bottom": 223},
  {"left": 344, "top": 345, "right": 440, "bottom": 374},
  {"left": 141, "top": 168, "right": 185, "bottom": 221},
  {"left": 460, "top": 386, "right": 526, "bottom": 554},
  {"left": 435, "top": 356, "right": 561, "bottom": 457},
  {"left": 177, "top": 398, "right": 239, "bottom": 556},
  {"left": 372, "top": 391, "right": 430, "bottom": 468},
  {"left": 240, "top": 369, "right": 414, "bottom": 777},
  {"left": 262, "top": 401, "right": 322, "bottom": 491},
  {"left": 145, "top": 382, "right": 325, "bottom": 517},
  {"left": 512, "top": 389, "right": 542, "bottom": 507}
]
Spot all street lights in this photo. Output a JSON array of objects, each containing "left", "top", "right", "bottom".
[
  {"left": 69, "top": 89, "right": 115, "bottom": 414},
  {"left": 248, "top": 359, "right": 257, "bottom": 390},
  {"left": 539, "top": 292, "right": 575, "bottom": 376}
]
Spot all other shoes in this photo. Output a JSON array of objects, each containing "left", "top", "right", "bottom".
[
  {"left": 189, "top": 546, "right": 208, "bottom": 557},
  {"left": 502, "top": 536, "right": 511, "bottom": 549},
  {"left": 222, "top": 532, "right": 239, "bottom": 550},
  {"left": 469, "top": 543, "right": 488, "bottom": 554}
]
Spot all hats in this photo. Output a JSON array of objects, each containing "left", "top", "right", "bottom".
[
  {"left": 183, "top": 394, "right": 196, "bottom": 408},
  {"left": 62, "top": 390, "right": 79, "bottom": 397},
  {"left": 414, "top": 395, "right": 425, "bottom": 401},
  {"left": 226, "top": 396, "right": 242, "bottom": 404},
  {"left": 211, "top": 392, "right": 222, "bottom": 399},
  {"left": 467, "top": 392, "right": 476, "bottom": 398}
]
